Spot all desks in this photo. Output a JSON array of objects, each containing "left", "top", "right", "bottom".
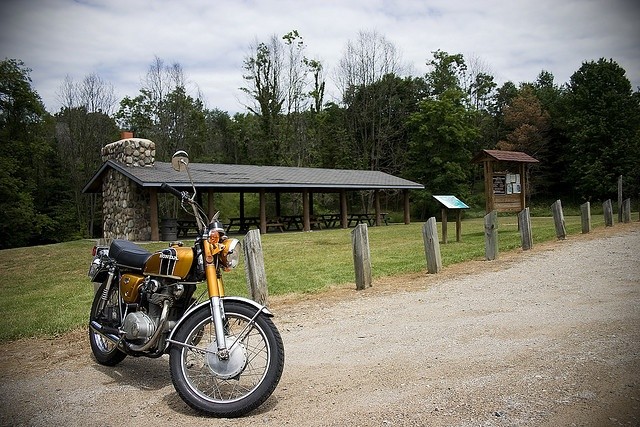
[
  {"left": 320, "top": 214, "right": 341, "bottom": 227},
  {"left": 348, "top": 212, "right": 389, "bottom": 226},
  {"left": 226, "top": 217, "right": 260, "bottom": 233},
  {"left": 176, "top": 219, "right": 202, "bottom": 237},
  {"left": 282, "top": 215, "right": 303, "bottom": 230}
]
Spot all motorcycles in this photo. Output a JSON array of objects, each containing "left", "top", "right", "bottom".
[{"left": 86, "top": 150, "right": 285, "bottom": 417}]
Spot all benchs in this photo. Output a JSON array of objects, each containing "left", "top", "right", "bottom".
[
  {"left": 372, "top": 218, "right": 390, "bottom": 225},
  {"left": 308, "top": 220, "right": 323, "bottom": 230},
  {"left": 181, "top": 225, "right": 200, "bottom": 237},
  {"left": 265, "top": 223, "right": 283, "bottom": 233},
  {"left": 348, "top": 218, "right": 372, "bottom": 226},
  {"left": 327, "top": 219, "right": 343, "bottom": 228}
]
